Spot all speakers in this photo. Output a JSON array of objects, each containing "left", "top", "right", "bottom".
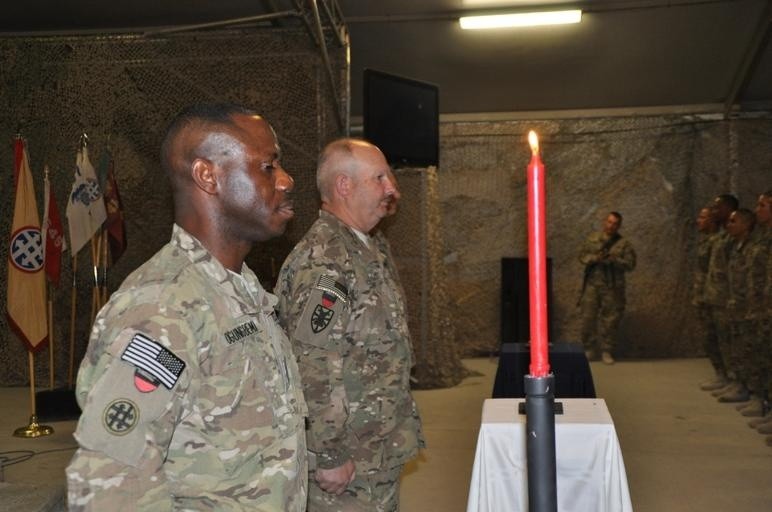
[
  {"left": 601, "top": 351, "right": 614, "bottom": 364},
  {"left": 585, "top": 350, "right": 596, "bottom": 362},
  {"left": 700, "top": 368, "right": 772, "bottom": 445}
]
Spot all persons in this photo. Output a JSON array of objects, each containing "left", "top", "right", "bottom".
[
  {"left": 367, "top": 169, "right": 418, "bottom": 369},
  {"left": 268, "top": 138, "right": 422, "bottom": 511},
  {"left": 571, "top": 211, "right": 636, "bottom": 365},
  {"left": 687, "top": 188, "right": 772, "bottom": 439},
  {"left": 62, "top": 99, "right": 308, "bottom": 510}
]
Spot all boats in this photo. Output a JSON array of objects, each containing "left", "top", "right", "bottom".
[{"left": 500, "top": 257, "right": 552, "bottom": 338}]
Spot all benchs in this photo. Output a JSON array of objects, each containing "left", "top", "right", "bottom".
[{"left": 8, "top": 133, "right": 128, "bottom": 354}]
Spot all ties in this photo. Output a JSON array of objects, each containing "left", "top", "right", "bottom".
[{"left": 362, "top": 68, "right": 439, "bottom": 169}]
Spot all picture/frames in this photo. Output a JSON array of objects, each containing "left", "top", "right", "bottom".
[
  {"left": 487, "top": 341, "right": 594, "bottom": 399},
  {"left": 468, "top": 395, "right": 630, "bottom": 510}
]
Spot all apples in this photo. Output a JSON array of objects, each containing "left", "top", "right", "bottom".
[{"left": 525, "top": 128, "right": 552, "bottom": 374}]
